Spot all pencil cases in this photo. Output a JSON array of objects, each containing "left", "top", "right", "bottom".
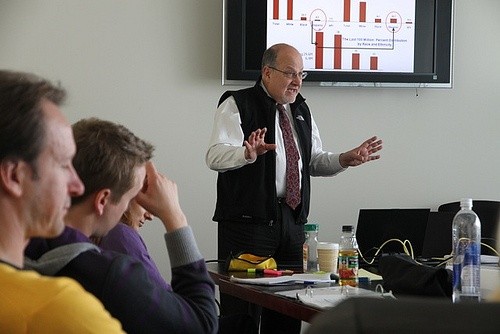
[{"left": 227, "top": 252, "right": 278, "bottom": 272}]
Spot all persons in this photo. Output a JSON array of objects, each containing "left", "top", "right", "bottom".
[
  {"left": 0, "top": 70, "right": 126, "bottom": 333},
  {"left": 23, "top": 117, "right": 218, "bottom": 333},
  {"left": 206, "top": 43, "right": 384, "bottom": 331}
]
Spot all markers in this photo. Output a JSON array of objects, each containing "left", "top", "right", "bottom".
[{"left": 247, "top": 268, "right": 294, "bottom": 275}]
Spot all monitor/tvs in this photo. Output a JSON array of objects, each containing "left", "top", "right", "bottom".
[{"left": 222, "top": 0, "right": 454, "bottom": 88}]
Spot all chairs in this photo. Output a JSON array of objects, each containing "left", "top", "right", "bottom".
[
  {"left": 438, "top": 200, "right": 499, "bottom": 260},
  {"left": 302, "top": 293, "right": 500, "bottom": 334}
]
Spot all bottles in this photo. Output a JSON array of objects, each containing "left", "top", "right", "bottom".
[
  {"left": 302, "top": 223, "right": 320, "bottom": 274},
  {"left": 452, "top": 198, "right": 481, "bottom": 303},
  {"left": 338, "top": 225, "right": 359, "bottom": 287}
]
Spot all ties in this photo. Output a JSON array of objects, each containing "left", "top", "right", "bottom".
[{"left": 276, "top": 103, "right": 301, "bottom": 210}]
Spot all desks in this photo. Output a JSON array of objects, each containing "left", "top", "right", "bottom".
[{"left": 203, "top": 257, "right": 379, "bottom": 334}]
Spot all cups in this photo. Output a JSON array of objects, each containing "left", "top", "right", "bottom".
[{"left": 317, "top": 242, "right": 339, "bottom": 274}]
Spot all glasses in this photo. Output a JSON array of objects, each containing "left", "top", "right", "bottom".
[{"left": 268, "top": 65, "right": 308, "bottom": 79}]
